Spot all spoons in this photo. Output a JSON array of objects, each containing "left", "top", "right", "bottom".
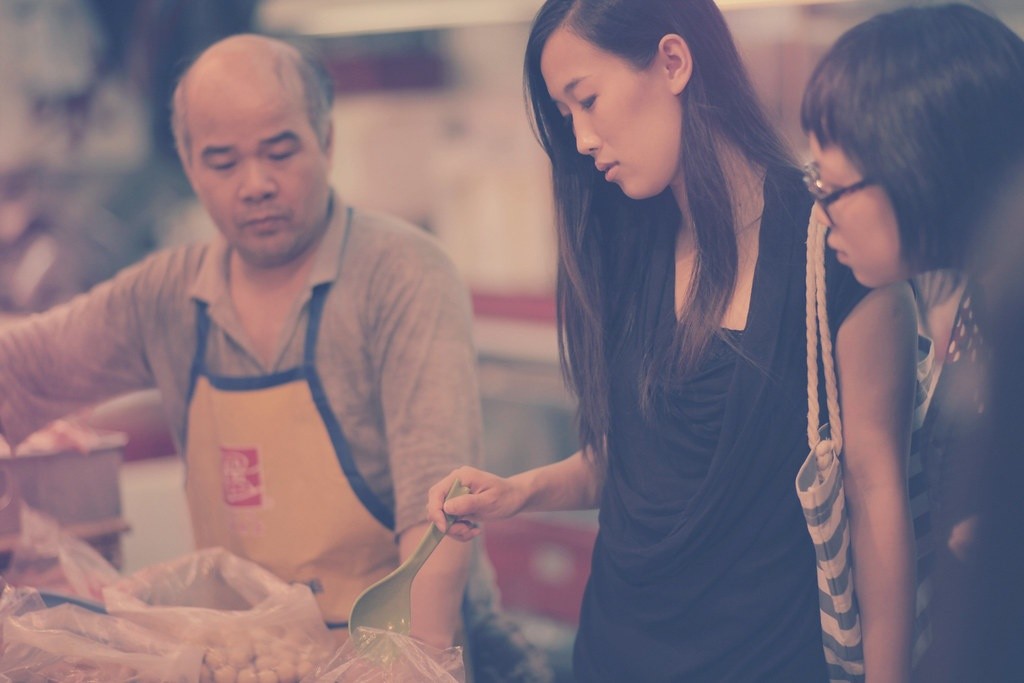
[{"left": 347, "top": 480, "right": 470, "bottom": 664}]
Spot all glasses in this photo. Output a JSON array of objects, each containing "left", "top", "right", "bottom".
[{"left": 802, "top": 163, "right": 873, "bottom": 229}]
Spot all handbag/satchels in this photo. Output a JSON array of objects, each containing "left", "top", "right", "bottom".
[{"left": 795, "top": 334, "right": 940, "bottom": 683}]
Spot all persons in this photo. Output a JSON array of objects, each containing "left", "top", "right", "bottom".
[
  {"left": 0, "top": 33, "right": 478, "bottom": 683},
  {"left": 428, "top": 0, "right": 1024, "bottom": 683}
]
